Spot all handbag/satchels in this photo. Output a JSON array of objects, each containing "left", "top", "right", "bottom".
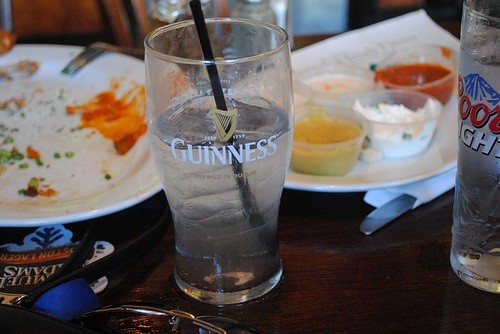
[{"left": 0, "top": 229, "right": 135, "bottom": 334}]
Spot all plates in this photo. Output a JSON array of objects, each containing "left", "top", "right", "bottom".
[
  {"left": 283, "top": 8, "right": 462, "bottom": 193},
  {"left": 1, "top": 43, "right": 193, "bottom": 227}
]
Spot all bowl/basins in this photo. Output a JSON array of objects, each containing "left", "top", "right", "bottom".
[{"left": 290, "top": 42, "right": 460, "bottom": 177}]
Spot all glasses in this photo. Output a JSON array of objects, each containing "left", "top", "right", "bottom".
[{"left": 70, "top": 300, "right": 265, "bottom": 334}]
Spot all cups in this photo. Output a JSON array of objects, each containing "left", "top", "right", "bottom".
[
  {"left": 449, "top": 0, "right": 500, "bottom": 294},
  {"left": 142, "top": 0, "right": 293, "bottom": 308}
]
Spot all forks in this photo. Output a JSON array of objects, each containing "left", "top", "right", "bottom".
[{"left": 62, "top": 42, "right": 144, "bottom": 75}]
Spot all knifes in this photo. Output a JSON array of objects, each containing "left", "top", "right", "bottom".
[{"left": 359, "top": 193, "right": 417, "bottom": 235}]
